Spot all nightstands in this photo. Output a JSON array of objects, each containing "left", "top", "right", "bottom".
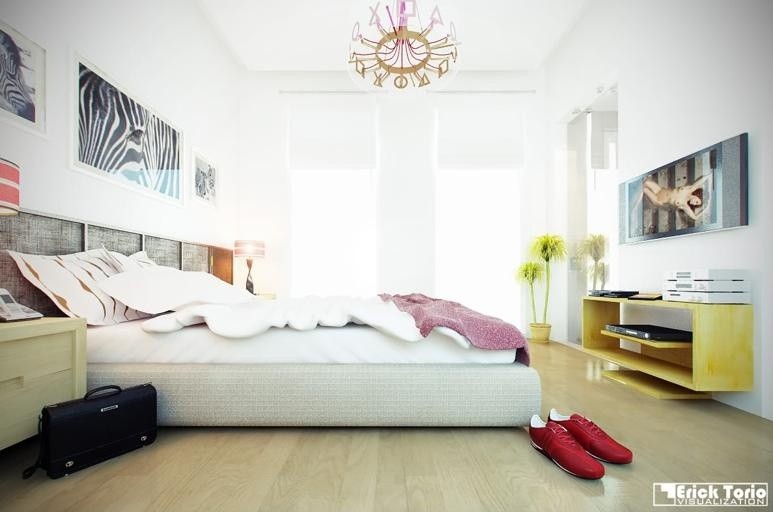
[{"left": 0, "top": 316, "right": 88, "bottom": 450}]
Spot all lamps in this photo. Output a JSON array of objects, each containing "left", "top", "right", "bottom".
[
  {"left": 0, "top": 157, "right": 23, "bottom": 216},
  {"left": 234, "top": 240, "right": 264, "bottom": 295},
  {"left": 347, "top": 0, "right": 463, "bottom": 96}
]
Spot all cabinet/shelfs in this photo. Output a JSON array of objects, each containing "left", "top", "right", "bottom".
[{"left": 582, "top": 293, "right": 755, "bottom": 400}]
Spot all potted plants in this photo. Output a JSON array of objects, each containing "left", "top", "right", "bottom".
[{"left": 518, "top": 232, "right": 566, "bottom": 344}]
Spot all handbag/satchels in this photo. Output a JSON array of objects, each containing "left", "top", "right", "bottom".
[{"left": 22, "top": 385, "right": 157, "bottom": 480}]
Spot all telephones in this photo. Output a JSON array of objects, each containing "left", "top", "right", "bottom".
[{"left": 0, "top": 287, "right": 44, "bottom": 322}]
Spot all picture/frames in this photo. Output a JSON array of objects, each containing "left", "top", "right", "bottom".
[
  {"left": 0, "top": 19, "right": 46, "bottom": 138},
  {"left": 69, "top": 50, "right": 185, "bottom": 208}
]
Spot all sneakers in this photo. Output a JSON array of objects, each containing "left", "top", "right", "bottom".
[
  {"left": 529, "top": 414, "right": 605, "bottom": 479},
  {"left": 548, "top": 409, "right": 633, "bottom": 465}
]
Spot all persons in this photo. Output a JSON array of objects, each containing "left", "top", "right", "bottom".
[{"left": 630, "top": 174, "right": 711, "bottom": 221}]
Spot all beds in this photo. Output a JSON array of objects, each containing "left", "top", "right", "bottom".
[{"left": 1, "top": 210, "right": 541, "bottom": 428}]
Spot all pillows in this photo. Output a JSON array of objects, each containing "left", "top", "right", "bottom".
[
  {"left": 111, "top": 250, "right": 172, "bottom": 270},
  {"left": 8, "top": 246, "right": 151, "bottom": 326},
  {"left": 99, "top": 266, "right": 250, "bottom": 315}
]
